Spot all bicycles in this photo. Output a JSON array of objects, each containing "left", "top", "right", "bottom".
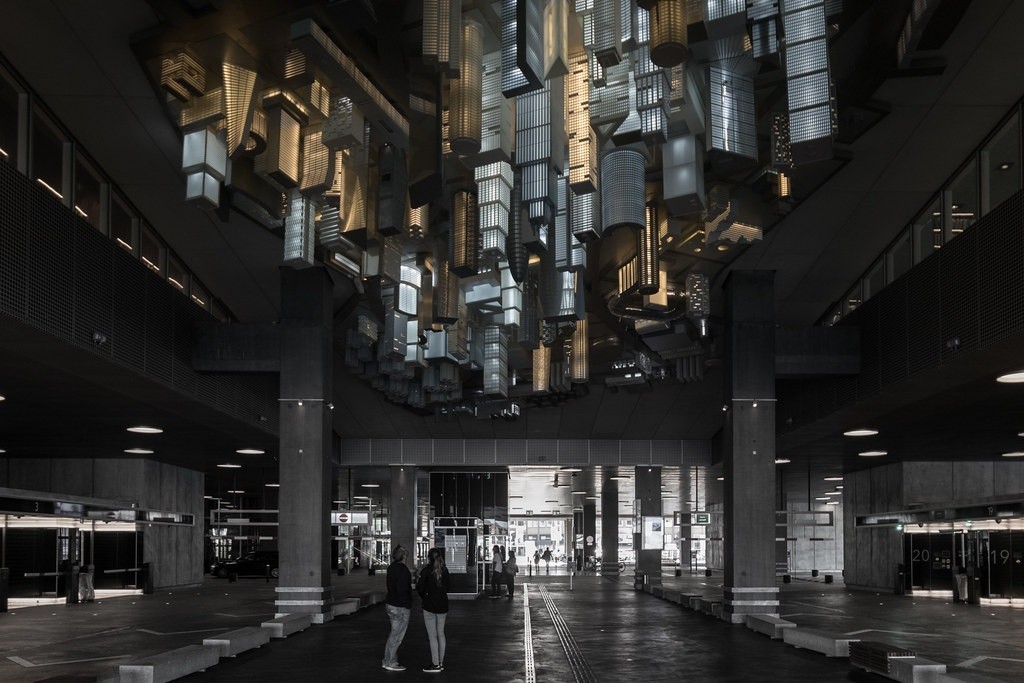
[{"left": 581, "top": 555, "right": 626, "bottom": 576}]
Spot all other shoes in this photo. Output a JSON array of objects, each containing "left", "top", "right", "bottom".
[
  {"left": 506, "top": 594, "right": 513, "bottom": 597},
  {"left": 488, "top": 594, "right": 497, "bottom": 598},
  {"left": 497, "top": 594, "right": 502, "bottom": 598}
]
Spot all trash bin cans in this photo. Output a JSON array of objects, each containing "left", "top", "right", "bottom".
[
  {"left": 141, "top": 562, "right": 154, "bottom": 595},
  {"left": 894, "top": 562, "right": 905, "bottom": 595},
  {"left": 66, "top": 563, "right": 95, "bottom": 604},
  {"left": 968, "top": 576, "right": 981, "bottom": 607}
]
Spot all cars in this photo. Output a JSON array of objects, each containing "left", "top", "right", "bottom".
[{"left": 209, "top": 549, "right": 280, "bottom": 581}]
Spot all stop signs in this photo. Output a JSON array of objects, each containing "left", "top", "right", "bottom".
[{"left": 339, "top": 513, "right": 348, "bottom": 522}]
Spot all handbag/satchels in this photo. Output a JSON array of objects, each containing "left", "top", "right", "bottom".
[{"left": 502, "top": 563, "right": 506, "bottom": 574}]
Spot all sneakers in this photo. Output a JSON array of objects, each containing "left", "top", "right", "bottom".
[
  {"left": 422, "top": 664, "right": 444, "bottom": 672},
  {"left": 382, "top": 663, "right": 407, "bottom": 671}
]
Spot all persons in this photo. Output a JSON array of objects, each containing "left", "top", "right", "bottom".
[
  {"left": 414, "top": 548, "right": 450, "bottom": 673},
  {"left": 478, "top": 545, "right": 561, "bottom": 599},
  {"left": 382, "top": 546, "right": 413, "bottom": 670}
]
